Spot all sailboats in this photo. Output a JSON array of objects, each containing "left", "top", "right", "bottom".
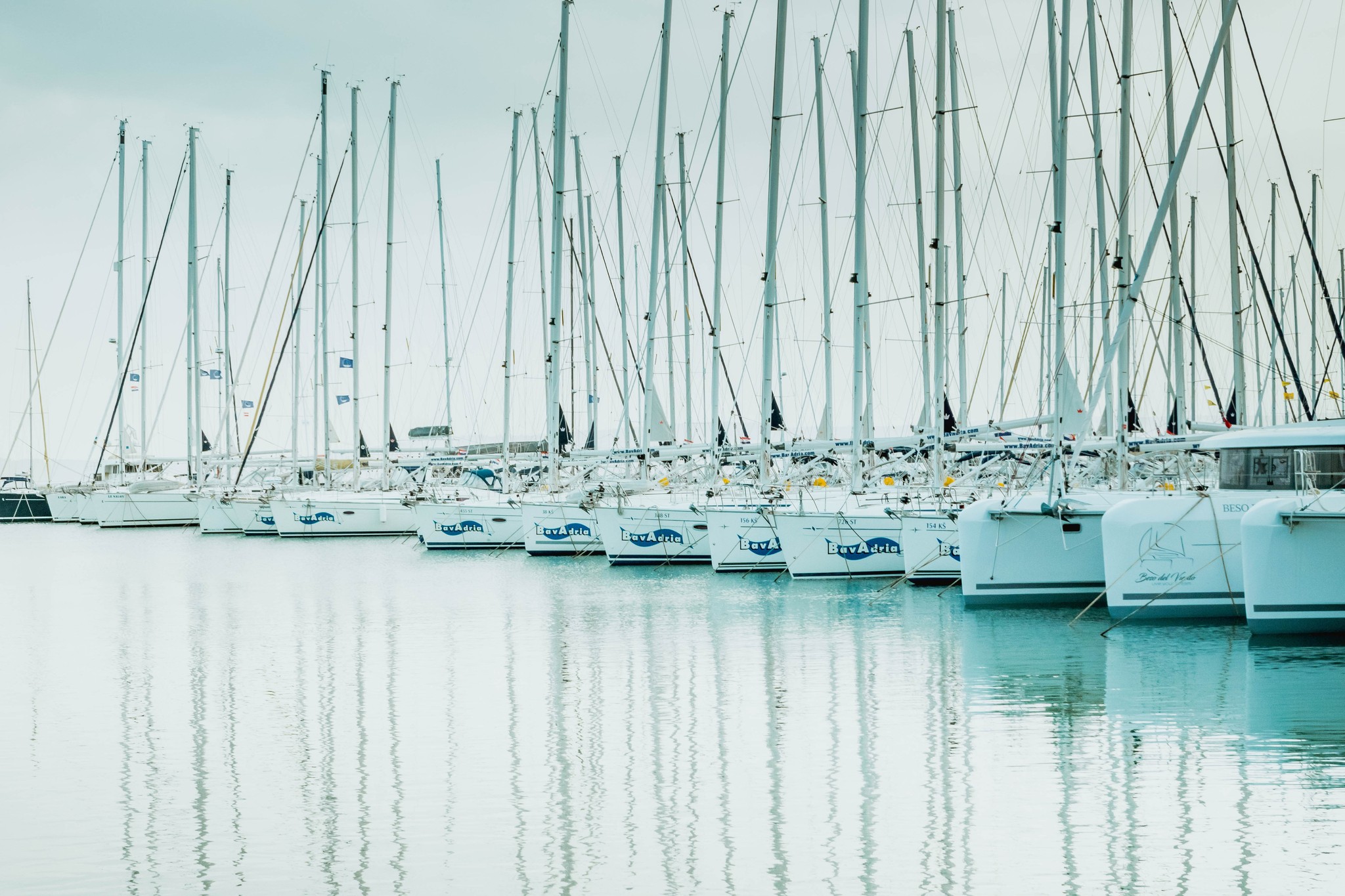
[{"left": 0, "top": 0, "right": 1343, "bottom": 651}]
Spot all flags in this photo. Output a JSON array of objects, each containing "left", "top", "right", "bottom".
[
  {"left": 589, "top": 395, "right": 600, "bottom": 403},
  {"left": 131, "top": 386, "right": 138, "bottom": 391},
  {"left": 340, "top": 357, "right": 353, "bottom": 368},
  {"left": 130, "top": 374, "right": 140, "bottom": 381},
  {"left": 241, "top": 400, "right": 254, "bottom": 408},
  {"left": 200, "top": 369, "right": 210, "bottom": 376},
  {"left": 210, "top": 369, "right": 222, "bottom": 379},
  {"left": 337, "top": 396, "right": 350, "bottom": 405}
]
